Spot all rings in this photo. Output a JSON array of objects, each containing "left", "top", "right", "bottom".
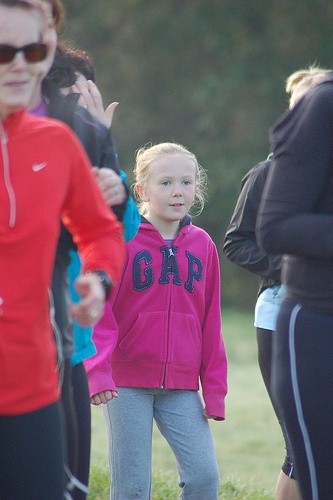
[{"left": 82, "top": 104, "right": 88, "bottom": 110}]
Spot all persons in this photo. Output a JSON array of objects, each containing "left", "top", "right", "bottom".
[
  {"left": 48, "top": 43, "right": 140, "bottom": 500},
  {"left": 0, "top": 0, "right": 128, "bottom": 500},
  {"left": 223, "top": 65, "right": 331, "bottom": 500},
  {"left": 28, "top": 0, "right": 125, "bottom": 500},
  {"left": 80, "top": 142, "right": 229, "bottom": 500},
  {"left": 255, "top": 79, "right": 333, "bottom": 497}
]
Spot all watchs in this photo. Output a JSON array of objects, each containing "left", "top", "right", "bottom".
[{"left": 88, "top": 269, "right": 112, "bottom": 299}]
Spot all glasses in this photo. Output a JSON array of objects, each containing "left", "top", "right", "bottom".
[{"left": 0, "top": 41, "right": 49, "bottom": 64}]
